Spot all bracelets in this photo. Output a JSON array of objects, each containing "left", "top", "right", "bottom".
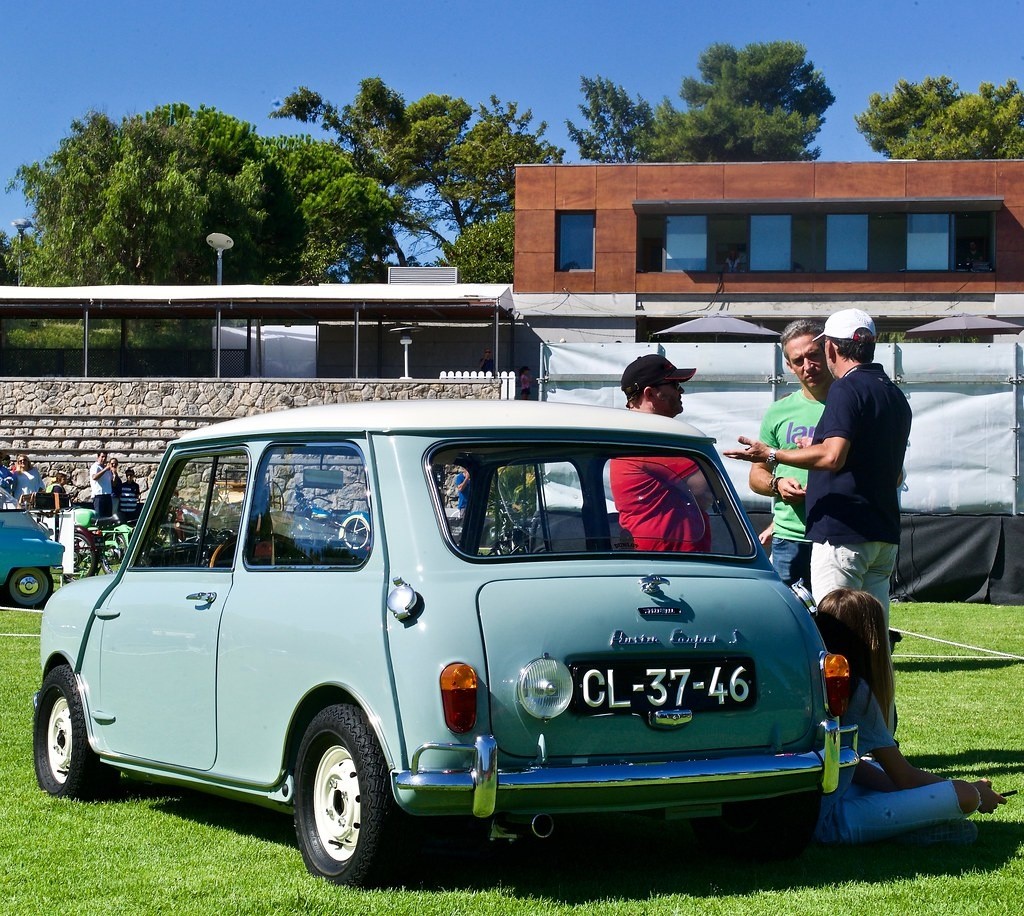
[
  {"left": 766, "top": 448, "right": 776, "bottom": 466},
  {"left": 770, "top": 476, "right": 785, "bottom": 496}
]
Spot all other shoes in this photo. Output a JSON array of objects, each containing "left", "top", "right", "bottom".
[{"left": 906, "top": 820, "right": 979, "bottom": 846}]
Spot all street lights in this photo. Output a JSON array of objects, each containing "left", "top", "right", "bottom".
[
  {"left": 206, "top": 232, "right": 234, "bottom": 285},
  {"left": 10, "top": 217, "right": 33, "bottom": 286}
]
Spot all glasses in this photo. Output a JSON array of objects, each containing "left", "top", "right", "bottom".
[
  {"left": 819, "top": 340, "right": 839, "bottom": 352},
  {"left": 19, "top": 459, "right": 27, "bottom": 462},
  {"left": 632, "top": 379, "right": 680, "bottom": 397}
]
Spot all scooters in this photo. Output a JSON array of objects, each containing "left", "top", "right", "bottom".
[{"left": 4, "top": 463, "right": 535, "bottom": 589}]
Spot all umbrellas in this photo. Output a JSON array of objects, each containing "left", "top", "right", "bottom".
[
  {"left": 653, "top": 318, "right": 781, "bottom": 343},
  {"left": 903, "top": 315, "right": 1024, "bottom": 343}
]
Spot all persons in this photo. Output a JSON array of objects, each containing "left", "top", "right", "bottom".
[
  {"left": 0, "top": 450, "right": 18, "bottom": 494},
  {"left": 814, "top": 590, "right": 1009, "bottom": 844},
  {"left": 722, "top": 308, "right": 912, "bottom": 636},
  {"left": 13, "top": 454, "right": 45, "bottom": 500},
  {"left": 45, "top": 471, "right": 67, "bottom": 493},
  {"left": 750, "top": 320, "right": 836, "bottom": 593},
  {"left": 478, "top": 348, "right": 496, "bottom": 376},
  {"left": 90, "top": 451, "right": 116, "bottom": 519},
  {"left": 9, "top": 462, "right": 16, "bottom": 474},
  {"left": 518, "top": 366, "right": 537, "bottom": 401},
  {"left": 454, "top": 467, "right": 470, "bottom": 517},
  {"left": 609, "top": 354, "right": 715, "bottom": 554},
  {"left": 120, "top": 469, "right": 141, "bottom": 519},
  {"left": 109, "top": 458, "right": 122, "bottom": 514},
  {"left": 964, "top": 240, "right": 983, "bottom": 269},
  {"left": 758, "top": 521, "right": 774, "bottom": 567}
]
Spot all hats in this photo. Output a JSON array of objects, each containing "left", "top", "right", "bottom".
[
  {"left": 621, "top": 354, "right": 696, "bottom": 391},
  {"left": 811, "top": 308, "right": 876, "bottom": 342}
]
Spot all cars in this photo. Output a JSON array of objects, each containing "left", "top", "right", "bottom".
[
  {"left": 0, "top": 484, "right": 66, "bottom": 608},
  {"left": 31, "top": 398, "right": 861, "bottom": 889}
]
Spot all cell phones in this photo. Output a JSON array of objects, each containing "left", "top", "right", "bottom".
[{"left": 1000, "top": 790, "right": 1018, "bottom": 797}]
